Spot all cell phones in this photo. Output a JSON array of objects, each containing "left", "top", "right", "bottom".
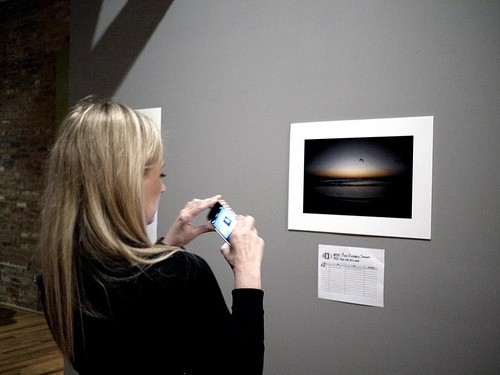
[{"left": 208, "top": 201, "right": 239, "bottom": 249}]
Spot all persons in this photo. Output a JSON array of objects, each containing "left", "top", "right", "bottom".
[{"left": 32, "top": 93, "right": 266, "bottom": 375}]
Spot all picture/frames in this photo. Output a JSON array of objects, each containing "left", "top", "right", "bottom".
[{"left": 287, "top": 115, "right": 433, "bottom": 241}]
